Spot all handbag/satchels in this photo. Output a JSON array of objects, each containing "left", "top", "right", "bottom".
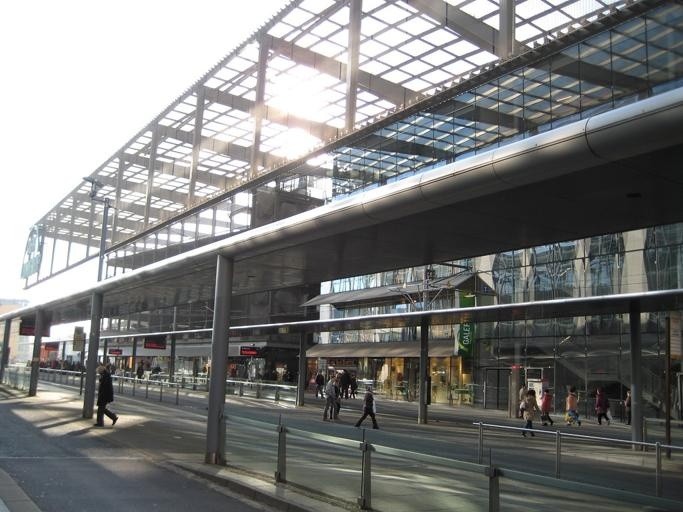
[
  {"left": 541, "top": 414, "right": 548, "bottom": 422},
  {"left": 565, "top": 411, "right": 576, "bottom": 422},
  {"left": 604, "top": 411, "right": 612, "bottom": 422}
]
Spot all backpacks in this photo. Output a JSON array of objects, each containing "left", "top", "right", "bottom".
[{"left": 520, "top": 401, "right": 529, "bottom": 411}]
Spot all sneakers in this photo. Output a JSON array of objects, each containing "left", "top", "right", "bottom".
[
  {"left": 112, "top": 417, "right": 118, "bottom": 426},
  {"left": 93, "top": 424, "right": 105, "bottom": 428},
  {"left": 598, "top": 420, "right": 610, "bottom": 425},
  {"left": 522, "top": 421, "right": 581, "bottom": 437}
]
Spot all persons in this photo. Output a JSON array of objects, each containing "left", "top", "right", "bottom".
[
  {"left": 623, "top": 391, "right": 631, "bottom": 425},
  {"left": 314, "top": 369, "right": 357, "bottom": 422},
  {"left": 93, "top": 366, "right": 118, "bottom": 427},
  {"left": 540, "top": 389, "right": 554, "bottom": 426},
  {"left": 354, "top": 386, "right": 379, "bottom": 430},
  {"left": 564, "top": 385, "right": 581, "bottom": 427},
  {"left": 595, "top": 386, "right": 611, "bottom": 426},
  {"left": 39, "top": 356, "right": 162, "bottom": 384},
  {"left": 517, "top": 384, "right": 542, "bottom": 437}
]
